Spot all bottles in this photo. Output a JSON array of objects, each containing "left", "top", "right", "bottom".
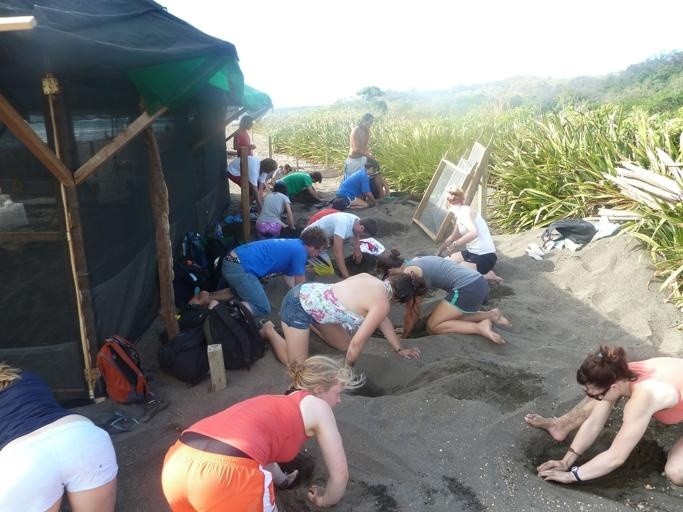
[
  {"left": 181, "top": 234, "right": 189, "bottom": 256},
  {"left": 214, "top": 221, "right": 223, "bottom": 240}
]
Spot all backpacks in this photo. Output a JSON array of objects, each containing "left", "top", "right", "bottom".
[
  {"left": 97, "top": 334, "right": 150, "bottom": 403},
  {"left": 541, "top": 219, "right": 596, "bottom": 252},
  {"left": 158, "top": 298, "right": 266, "bottom": 384},
  {"left": 174, "top": 230, "right": 225, "bottom": 308}
]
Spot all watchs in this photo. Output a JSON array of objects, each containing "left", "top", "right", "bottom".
[{"left": 569, "top": 465, "right": 583, "bottom": 484}]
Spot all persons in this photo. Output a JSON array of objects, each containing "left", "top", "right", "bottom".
[
  {"left": 523, "top": 345, "right": 682, "bottom": 488},
  {"left": 185, "top": 225, "right": 328, "bottom": 319},
  {"left": 257, "top": 272, "right": 428, "bottom": 371},
  {"left": 224, "top": 112, "right": 391, "bottom": 279},
  {"left": 374, "top": 249, "right": 513, "bottom": 345},
  {"left": 0, "top": 363, "right": 119, "bottom": 511},
  {"left": 160, "top": 353, "right": 351, "bottom": 511},
  {"left": 434, "top": 185, "right": 505, "bottom": 282}
]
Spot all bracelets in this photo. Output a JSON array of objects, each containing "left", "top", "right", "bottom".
[
  {"left": 394, "top": 346, "right": 405, "bottom": 354},
  {"left": 451, "top": 239, "right": 457, "bottom": 250},
  {"left": 565, "top": 446, "right": 581, "bottom": 457}
]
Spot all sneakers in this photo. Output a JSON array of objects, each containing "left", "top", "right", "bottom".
[{"left": 224, "top": 203, "right": 257, "bottom": 224}]
